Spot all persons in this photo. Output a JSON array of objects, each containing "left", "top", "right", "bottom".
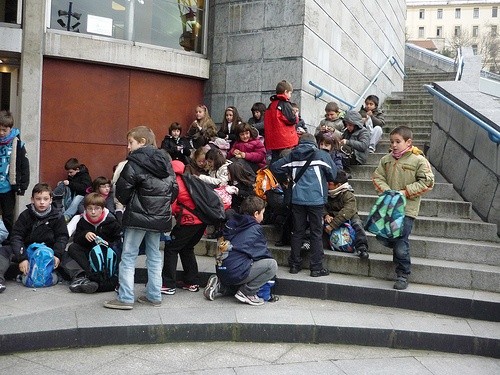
[
  {"left": 103, "top": 126, "right": 179, "bottom": 310},
  {"left": 372, "top": 125, "right": 435, "bottom": 290},
  {"left": 271, "top": 132, "right": 338, "bottom": 277},
  {"left": 0, "top": 158, "right": 128, "bottom": 294},
  {"left": 159, "top": 95, "right": 386, "bottom": 259},
  {"left": 262, "top": 79, "right": 299, "bottom": 168},
  {"left": 203, "top": 196, "right": 279, "bottom": 306},
  {"left": 160, "top": 152, "right": 206, "bottom": 295},
  {"left": 0, "top": 109, "right": 30, "bottom": 234}
]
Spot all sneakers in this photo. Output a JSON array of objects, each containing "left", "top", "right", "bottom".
[
  {"left": 393, "top": 273, "right": 410, "bottom": 290},
  {"left": 203, "top": 274, "right": 221, "bottom": 301},
  {"left": 356, "top": 250, "right": 369, "bottom": 258},
  {"left": 234, "top": 290, "right": 265, "bottom": 306}
]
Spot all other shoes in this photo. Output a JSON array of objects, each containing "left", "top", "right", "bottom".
[
  {"left": 0, "top": 280, "right": 6, "bottom": 293},
  {"left": 368, "top": 146, "right": 375, "bottom": 153},
  {"left": 176, "top": 280, "right": 200, "bottom": 292},
  {"left": 138, "top": 296, "right": 163, "bottom": 306},
  {"left": 301, "top": 243, "right": 310, "bottom": 250},
  {"left": 275, "top": 240, "right": 286, "bottom": 247},
  {"left": 311, "top": 268, "right": 329, "bottom": 277},
  {"left": 161, "top": 285, "right": 176, "bottom": 295},
  {"left": 103, "top": 299, "right": 134, "bottom": 310},
  {"left": 69, "top": 275, "right": 98, "bottom": 293},
  {"left": 289, "top": 264, "right": 302, "bottom": 274}
]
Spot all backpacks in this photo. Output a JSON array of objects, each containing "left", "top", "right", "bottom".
[
  {"left": 363, "top": 189, "right": 407, "bottom": 241},
  {"left": 86, "top": 242, "right": 119, "bottom": 292},
  {"left": 21, "top": 241, "right": 59, "bottom": 288},
  {"left": 175, "top": 172, "right": 226, "bottom": 226},
  {"left": 51, "top": 181, "right": 76, "bottom": 214},
  {"left": 329, "top": 219, "right": 356, "bottom": 253},
  {"left": 263, "top": 188, "right": 284, "bottom": 212},
  {"left": 253, "top": 168, "right": 278, "bottom": 200}
]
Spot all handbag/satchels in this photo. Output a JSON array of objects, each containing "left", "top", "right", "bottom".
[{"left": 284, "top": 179, "right": 295, "bottom": 210}]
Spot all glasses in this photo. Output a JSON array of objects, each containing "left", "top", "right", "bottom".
[
  {"left": 85, "top": 207, "right": 102, "bottom": 212},
  {"left": 100, "top": 186, "right": 110, "bottom": 188}
]
